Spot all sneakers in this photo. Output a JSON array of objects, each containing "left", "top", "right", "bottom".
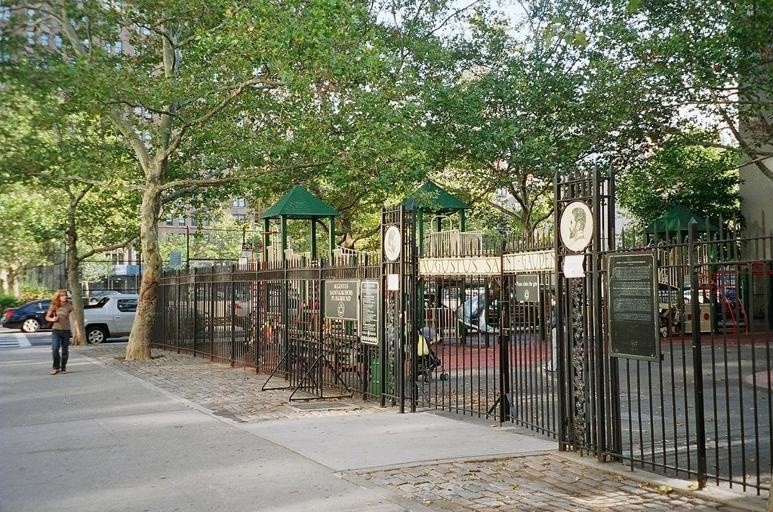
[
  {"left": 50, "top": 369, "right": 60, "bottom": 375},
  {"left": 61, "top": 371, "right": 66, "bottom": 374}
]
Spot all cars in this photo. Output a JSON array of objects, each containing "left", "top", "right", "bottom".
[
  {"left": 227, "top": 291, "right": 308, "bottom": 327},
  {"left": 0, "top": 299, "right": 56, "bottom": 333},
  {"left": 88, "top": 290, "right": 121, "bottom": 302}
]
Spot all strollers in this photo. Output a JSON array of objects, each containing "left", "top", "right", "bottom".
[{"left": 409, "top": 318, "right": 448, "bottom": 382}]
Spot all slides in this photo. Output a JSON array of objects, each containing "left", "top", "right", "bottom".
[{"left": 452, "top": 289, "right": 501, "bottom": 336}]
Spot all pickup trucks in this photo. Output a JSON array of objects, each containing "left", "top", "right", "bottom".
[{"left": 68, "top": 293, "right": 207, "bottom": 345}]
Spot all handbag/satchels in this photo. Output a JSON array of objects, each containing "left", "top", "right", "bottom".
[{"left": 45, "top": 311, "right": 54, "bottom": 328}]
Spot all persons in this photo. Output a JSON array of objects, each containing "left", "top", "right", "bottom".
[
  {"left": 45, "top": 290, "right": 80, "bottom": 374},
  {"left": 543, "top": 292, "right": 557, "bottom": 372},
  {"left": 386, "top": 229, "right": 400, "bottom": 261},
  {"left": 569, "top": 207, "right": 590, "bottom": 251}
]
